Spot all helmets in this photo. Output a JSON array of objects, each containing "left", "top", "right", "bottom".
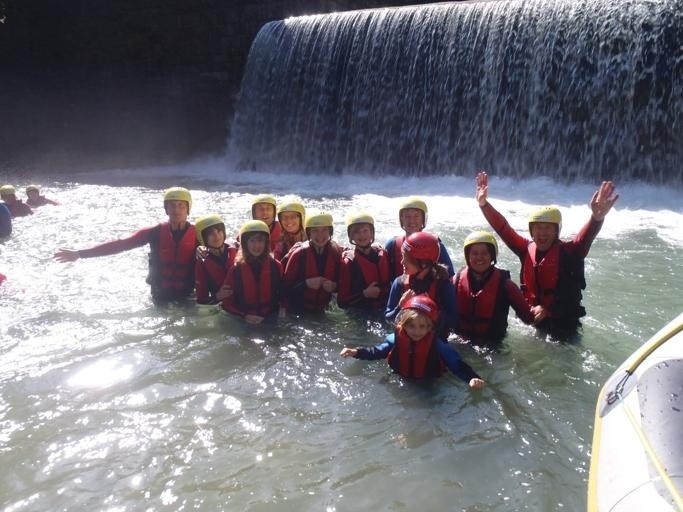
[
  {"left": 463, "top": 230, "right": 499, "bottom": 268},
  {"left": 400, "top": 232, "right": 442, "bottom": 265},
  {"left": 402, "top": 294, "right": 440, "bottom": 325},
  {"left": 251, "top": 195, "right": 276, "bottom": 220},
  {"left": 26, "top": 184, "right": 39, "bottom": 196},
  {"left": 304, "top": 210, "right": 334, "bottom": 243},
  {"left": 347, "top": 214, "right": 375, "bottom": 239},
  {"left": 236, "top": 220, "right": 271, "bottom": 250},
  {"left": 195, "top": 213, "right": 228, "bottom": 248},
  {"left": 528, "top": 206, "right": 563, "bottom": 240},
  {"left": 163, "top": 186, "right": 192, "bottom": 216},
  {"left": 277, "top": 201, "right": 306, "bottom": 232},
  {"left": 398, "top": 198, "right": 429, "bottom": 229},
  {"left": 0, "top": 184, "right": 16, "bottom": 197}
]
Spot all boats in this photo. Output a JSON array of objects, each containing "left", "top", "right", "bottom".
[{"left": 586, "top": 312, "right": 683, "bottom": 512}]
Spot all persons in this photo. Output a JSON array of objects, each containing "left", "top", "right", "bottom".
[
  {"left": 221, "top": 219, "right": 285, "bottom": 326},
  {"left": 53, "top": 187, "right": 199, "bottom": 307},
  {"left": 283, "top": 212, "right": 343, "bottom": 317},
  {"left": 195, "top": 194, "right": 281, "bottom": 261},
  {"left": 385, "top": 198, "right": 456, "bottom": 285},
  {"left": 384, "top": 231, "right": 458, "bottom": 343},
  {"left": 340, "top": 295, "right": 484, "bottom": 388},
  {"left": 25, "top": 184, "right": 60, "bottom": 209},
  {"left": 475, "top": 170, "right": 619, "bottom": 345},
  {"left": 335, "top": 212, "right": 388, "bottom": 314},
  {"left": 194, "top": 215, "right": 240, "bottom": 305},
  {"left": 432, "top": 231, "right": 547, "bottom": 346},
  {"left": 273, "top": 201, "right": 305, "bottom": 269},
  {"left": 0, "top": 184, "right": 34, "bottom": 217}
]
